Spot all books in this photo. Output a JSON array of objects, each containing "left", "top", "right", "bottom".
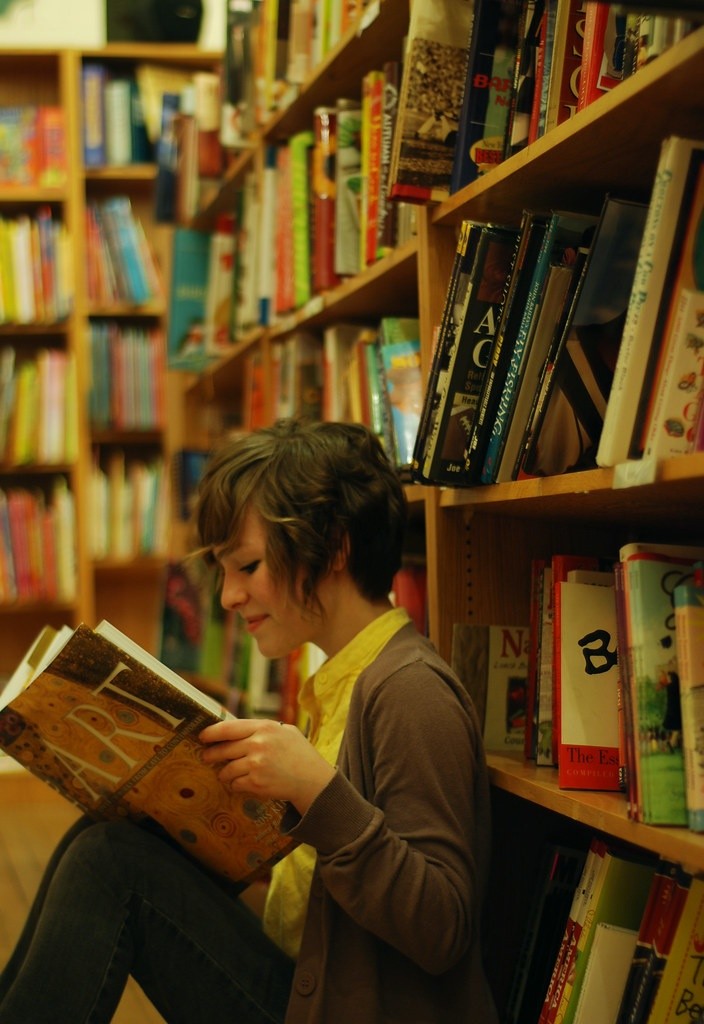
[
  {"left": 0, "top": 619, "right": 305, "bottom": 896},
  {"left": 0, "top": 1, "right": 703, "bottom": 1022}
]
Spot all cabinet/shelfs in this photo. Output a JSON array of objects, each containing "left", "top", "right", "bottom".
[{"left": 0, "top": 2, "right": 704, "bottom": 1024}]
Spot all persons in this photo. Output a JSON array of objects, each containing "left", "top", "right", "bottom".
[{"left": 0, "top": 415, "right": 487, "bottom": 1024}]
